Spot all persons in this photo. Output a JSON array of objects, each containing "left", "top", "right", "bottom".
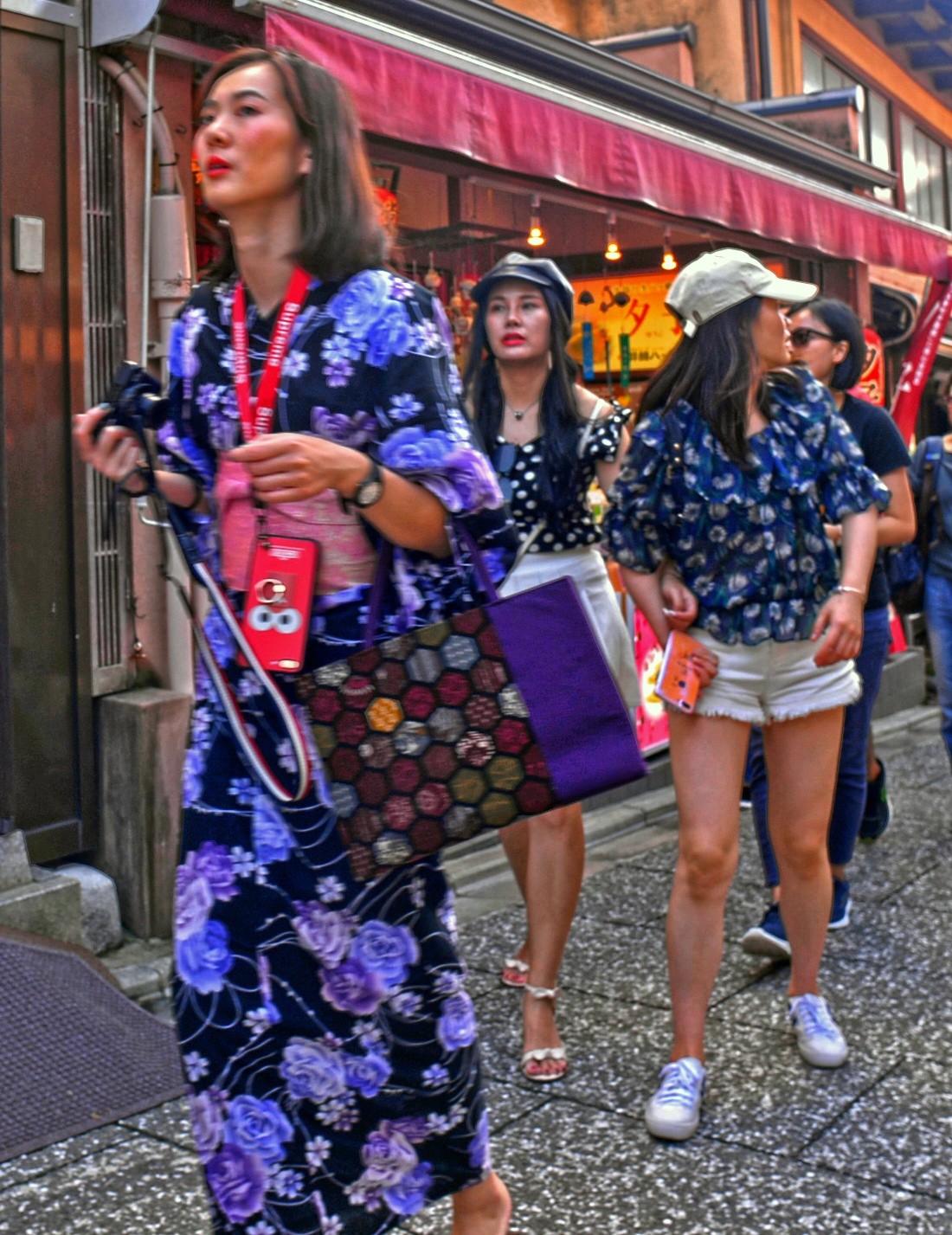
[
  {"left": 458, "top": 252, "right": 697, "bottom": 1081},
  {"left": 601, "top": 248, "right": 893, "bottom": 1140},
  {"left": 740, "top": 298, "right": 952, "bottom": 958},
  {"left": 75, "top": 48, "right": 520, "bottom": 1235}
]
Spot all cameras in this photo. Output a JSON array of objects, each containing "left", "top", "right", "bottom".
[{"left": 90, "top": 359, "right": 170, "bottom": 442}]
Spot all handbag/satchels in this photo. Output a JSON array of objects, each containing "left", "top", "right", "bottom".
[
  {"left": 887, "top": 438, "right": 941, "bottom": 612},
  {"left": 305, "top": 517, "right": 650, "bottom": 881}
]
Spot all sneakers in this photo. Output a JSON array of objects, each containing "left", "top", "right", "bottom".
[
  {"left": 645, "top": 1056, "right": 706, "bottom": 1140},
  {"left": 786, "top": 991, "right": 848, "bottom": 1067},
  {"left": 740, "top": 902, "right": 791, "bottom": 957},
  {"left": 826, "top": 876, "right": 854, "bottom": 929}
]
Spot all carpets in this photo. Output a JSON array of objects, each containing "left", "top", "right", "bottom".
[{"left": 0, "top": 925, "right": 185, "bottom": 1163}]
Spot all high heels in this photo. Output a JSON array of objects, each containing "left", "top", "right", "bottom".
[
  {"left": 520, "top": 984, "right": 567, "bottom": 1082},
  {"left": 501, "top": 955, "right": 532, "bottom": 987}
]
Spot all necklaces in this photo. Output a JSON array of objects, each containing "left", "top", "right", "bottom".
[{"left": 505, "top": 398, "right": 539, "bottom": 420}]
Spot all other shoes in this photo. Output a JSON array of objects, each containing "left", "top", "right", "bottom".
[
  {"left": 738, "top": 781, "right": 752, "bottom": 810},
  {"left": 858, "top": 758, "right": 894, "bottom": 846}
]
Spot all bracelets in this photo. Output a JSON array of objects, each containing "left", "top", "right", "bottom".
[
  {"left": 834, "top": 586, "right": 867, "bottom": 603},
  {"left": 172, "top": 476, "right": 203, "bottom": 512}
]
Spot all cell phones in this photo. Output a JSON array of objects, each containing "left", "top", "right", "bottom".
[{"left": 653, "top": 629, "right": 702, "bottom": 715}]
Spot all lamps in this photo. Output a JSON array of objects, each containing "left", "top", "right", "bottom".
[{"left": 526, "top": 193, "right": 677, "bottom": 271}]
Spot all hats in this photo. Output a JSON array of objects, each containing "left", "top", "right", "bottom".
[
  {"left": 664, "top": 247, "right": 820, "bottom": 338},
  {"left": 469, "top": 249, "right": 575, "bottom": 322}
]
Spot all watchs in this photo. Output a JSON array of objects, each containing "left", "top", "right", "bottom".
[{"left": 337, "top": 457, "right": 386, "bottom": 517}]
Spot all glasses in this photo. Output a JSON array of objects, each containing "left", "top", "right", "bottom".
[{"left": 789, "top": 326, "right": 840, "bottom": 347}]
